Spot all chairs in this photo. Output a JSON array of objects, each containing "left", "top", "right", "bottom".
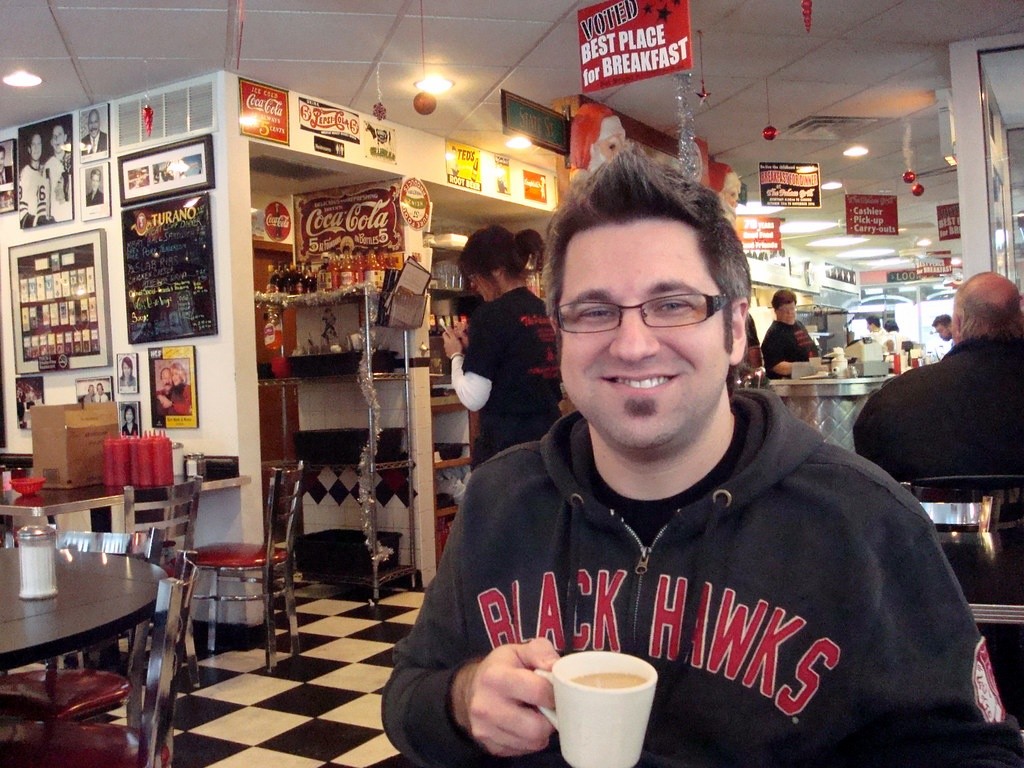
[{"left": 0, "top": 460, "right": 306, "bottom": 768}]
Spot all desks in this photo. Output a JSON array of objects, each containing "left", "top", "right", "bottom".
[
  {"left": 0, "top": 547, "right": 168, "bottom": 673},
  {"left": 0, "top": 475, "right": 252, "bottom": 533}
]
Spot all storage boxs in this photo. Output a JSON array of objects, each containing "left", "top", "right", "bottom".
[
  {"left": 294, "top": 529, "right": 403, "bottom": 576},
  {"left": 292, "top": 428, "right": 402, "bottom": 464},
  {"left": 287, "top": 350, "right": 399, "bottom": 377},
  {"left": 30, "top": 403, "right": 120, "bottom": 489}
]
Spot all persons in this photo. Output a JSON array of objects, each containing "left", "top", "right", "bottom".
[
  {"left": 0, "top": 107, "right": 189, "bottom": 432},
  {"left": 735, "top": 270, "right": 1024, "bottom": 527},
  {"left": 435, "top": 224, "right": 566, "bottom": 478},
  {"left": 382, "top": 156, "right": 1023, "bottom": 768}
]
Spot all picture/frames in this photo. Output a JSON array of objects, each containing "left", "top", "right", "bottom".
[
  {"left": 117, "top": 133, "right": 215, "bottom": 206},
  {"left": 75, "top": 353, "right": 142, "bottom": 438}
]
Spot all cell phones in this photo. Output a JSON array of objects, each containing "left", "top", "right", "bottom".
[{"left": 439, "top": 319, "right": 451, "bottom": 336}]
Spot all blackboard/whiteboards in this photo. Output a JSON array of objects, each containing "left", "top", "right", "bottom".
[{"left": 121, "top": 190, "right": 218, "bottom": 344}]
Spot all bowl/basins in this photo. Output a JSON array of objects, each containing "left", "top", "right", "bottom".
[{"left": 9, "top": 477, "right": 46, "bottom": 495}]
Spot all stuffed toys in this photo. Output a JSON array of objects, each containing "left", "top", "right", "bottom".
[{"left": 565, "top": 106, "right": 742, "bottom": 224}]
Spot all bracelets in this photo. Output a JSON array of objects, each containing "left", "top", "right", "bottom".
[{"left": 450, "top": 353, "right": 464, "bottom": 360}]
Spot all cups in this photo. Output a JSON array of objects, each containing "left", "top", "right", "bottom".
[{"left": 533, "top": 651, "right": 657, "bottom": 768}]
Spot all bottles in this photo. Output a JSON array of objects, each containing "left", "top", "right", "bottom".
[
  {"left": 263, "top": 308, "right": 283, "bottom": 352},
  {"left": 102, "top": 430, "right": 206, "bottom": 487},
  {"left": 264, "top": 245, "right": 399, "bottom": 295},
  {"left": 15, "top": 524, "right": 58, "bottom": 600},
  {"left": 831, "top": 354, "right": 849, "bottom": 379}
]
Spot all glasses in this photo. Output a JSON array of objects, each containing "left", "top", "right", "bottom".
[
  {"left": 468, "top": 270, "right": 484, "bottom": 282},
  {"left": 557, "top": 294, "right": 733, "bottom": 334},
  {"left": 777, "top": 307, "right": 797, "bottom": 313}
]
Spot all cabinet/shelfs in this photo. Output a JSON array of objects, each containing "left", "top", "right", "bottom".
[{"left": 256, "top": 288, "right": 476, "bottom": 603}]
